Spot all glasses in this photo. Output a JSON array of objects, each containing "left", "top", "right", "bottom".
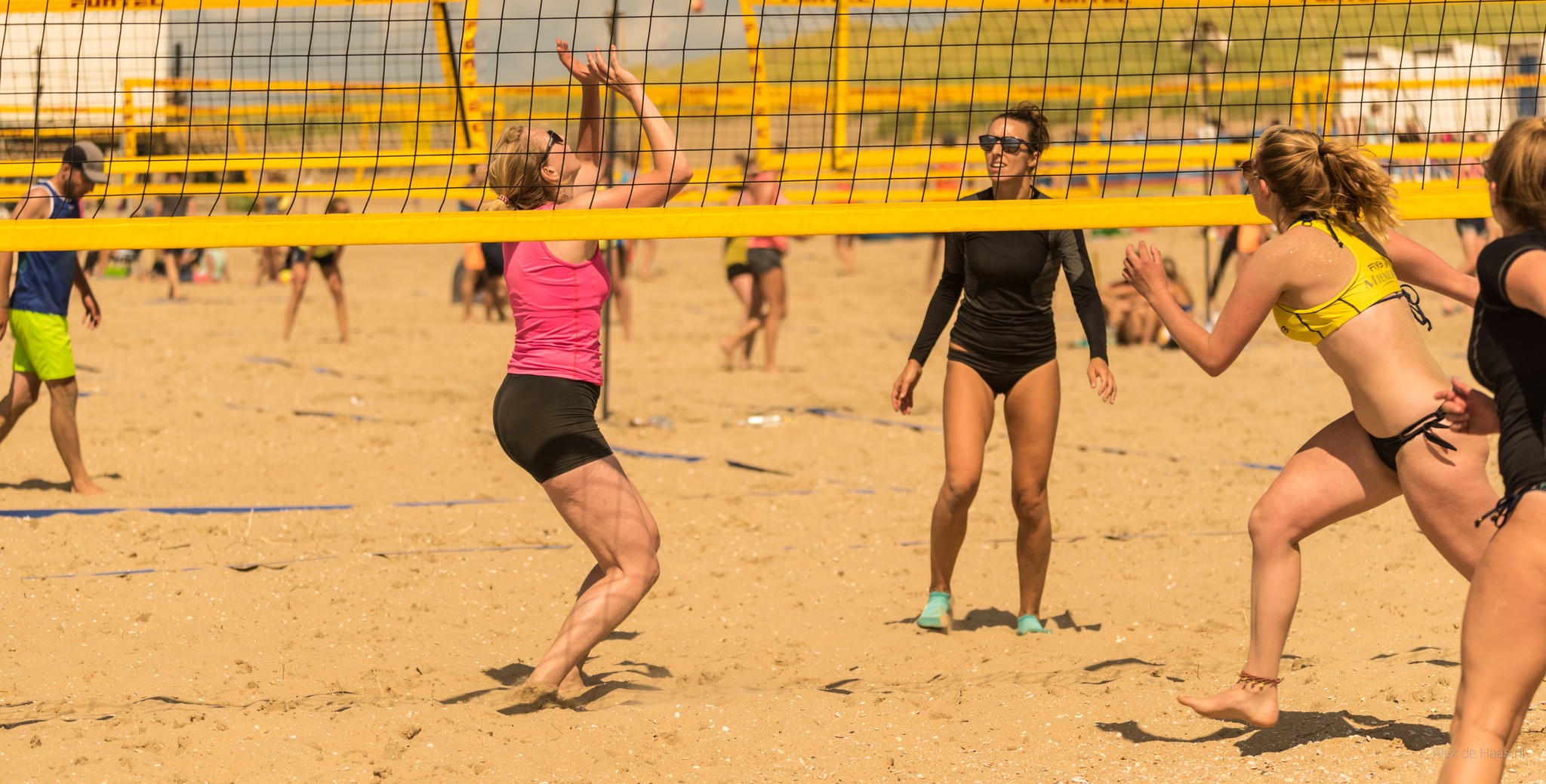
[
  {"left": 978, "top": 134, "right": 1034, "bottom": 154},
  {"left": 539, "top": 129, "right": 563, "bottom": 169},
  {"left": 1481, "top": 159, "right": 1503, "bottom": 185},
  {"left": 1239, "top": 158, "right": 1284, "bottom": 193}
]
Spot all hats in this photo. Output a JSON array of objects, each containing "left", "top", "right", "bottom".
[{"left": 62, "top": 140, "right": 112, "bottom": 184}]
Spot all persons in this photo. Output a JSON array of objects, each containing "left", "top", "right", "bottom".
[
  {"left": 474, "top": 41, "right": 687, "bottom": 694},
  {"left": 2, "top": 142, "right": 1512, "bottom": 497},
  {"left": 1125, "top": 126, "right": 1509, "bottom": 729},
  {"left": 1431, "top": 115, "right": 1546, "bottom": 784},
  {"left": 890, "top": 106, "right": 1117, "bottom": 642}
]
